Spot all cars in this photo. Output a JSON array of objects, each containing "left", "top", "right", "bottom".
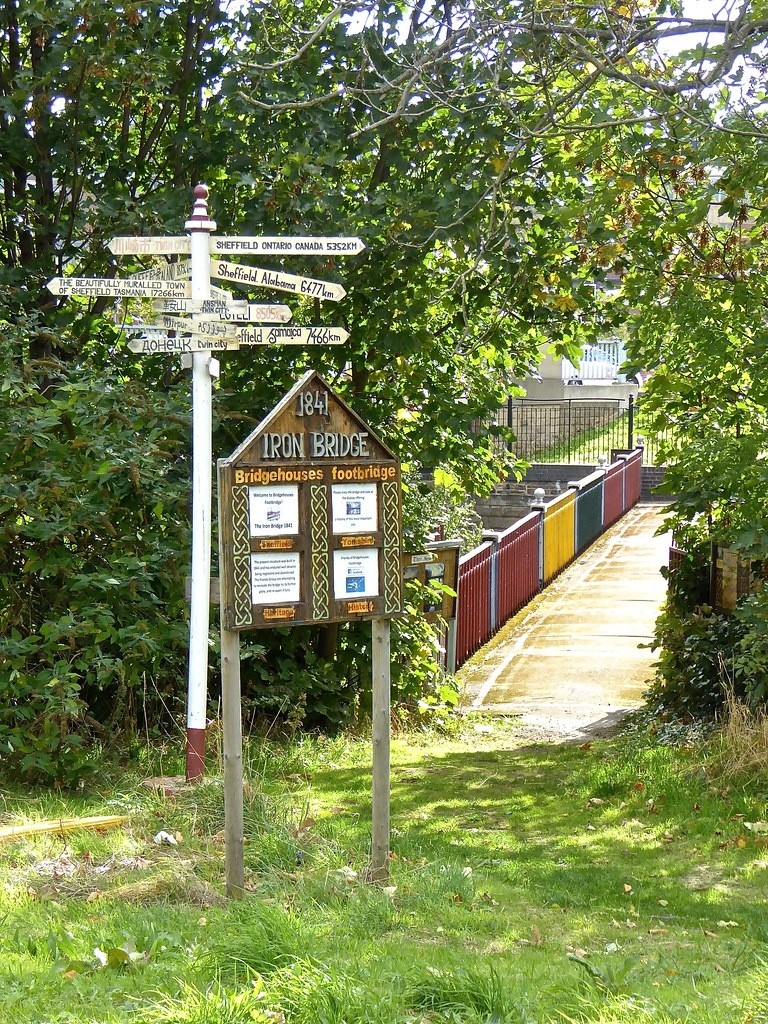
[{"left": 562, "top": 344, "right": 643, "bottom": 389}]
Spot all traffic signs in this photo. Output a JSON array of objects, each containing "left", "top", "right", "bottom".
[
  {"left": 210, "top": 259, "right": 347, "bottom": 303},
  {"left": 152, "top": 298, "right": 249, "bottom": 316},
  {"left": 210, "top": 284, "right": 233, "bottom": 301},
  {"left": 237, "top": 326, "right": 352, "bottom": 346},
  {"left": 45, "top": 276, "right": 193, "bottom": 299},
  {"left": 209, "top": 234, "right": 367, "bottom": 255},
  {"left": 127, "top": 337, "right": 240, "bottom": 353},
  {"left": 107, "top": 235, "right": 192, "bottom": 255},
  {"left": 156, "top": 314, "right": 238, "bottom": 337},
  {"left": 212, "top": 304, "right": 294, "bottom": 324},
  {"left": 129, "top": 258, "right": 192, "bottom": 281}
]
